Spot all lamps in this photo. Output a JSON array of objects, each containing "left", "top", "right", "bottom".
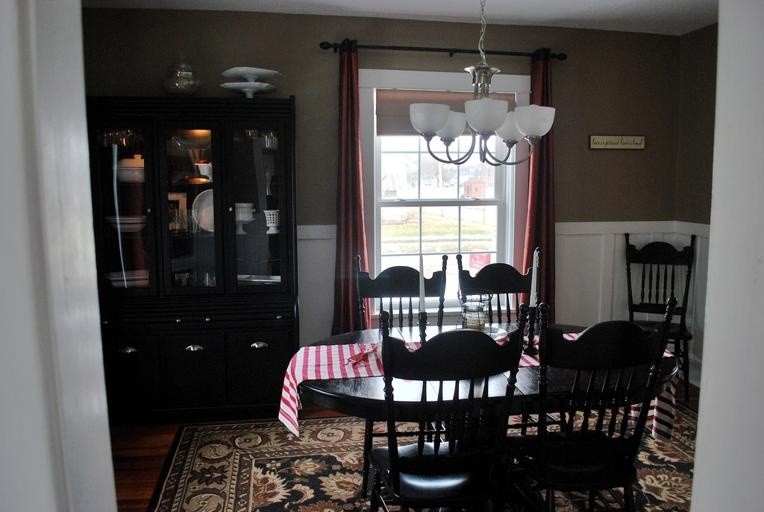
[{"left": 410, "top": 0, "right": 556, "bottom": 166}]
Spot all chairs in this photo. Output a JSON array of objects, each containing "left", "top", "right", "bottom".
[{"left": 624, "top": 233, "right": 697, "bottom": 401}]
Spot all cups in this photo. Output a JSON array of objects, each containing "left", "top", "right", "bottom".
[{"left": 461, "top": 301, "right": 487, "bottom": 333}]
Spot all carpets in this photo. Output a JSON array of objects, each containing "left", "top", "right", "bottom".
[{"left": 145, "top": 403, "right": 698, "bottom": 512}]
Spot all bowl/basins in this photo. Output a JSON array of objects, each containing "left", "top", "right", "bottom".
[{"left": 106, "top": 214, "right": 147, "bottom": 233}]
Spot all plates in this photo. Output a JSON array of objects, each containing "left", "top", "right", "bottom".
[{"left": 191, "top": 189, "right": 214, "bottom": 234}]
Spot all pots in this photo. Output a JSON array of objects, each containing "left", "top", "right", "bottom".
[{"left": 114, "top": 154, "right": 145, "bottom": 184}]
